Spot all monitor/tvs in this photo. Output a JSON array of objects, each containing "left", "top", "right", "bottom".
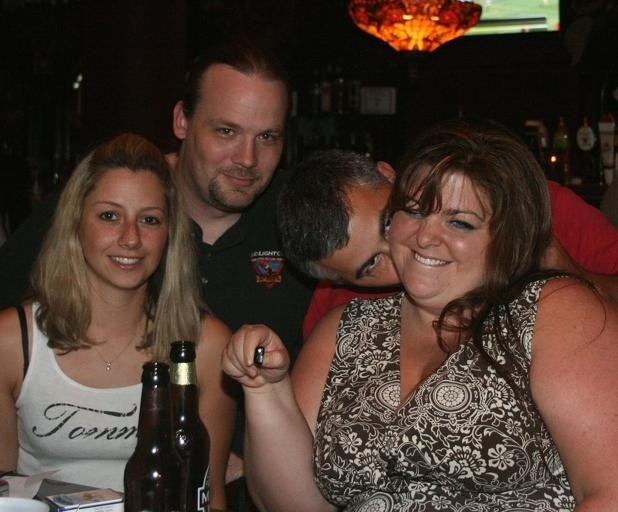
[{"left": 463, "top": 0, "right": 565, "bottom": 39}]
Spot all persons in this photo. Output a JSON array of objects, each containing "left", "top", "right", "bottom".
[
  {"left": 162, "top": 49, "right": 318, "bottom": 511},
  {"left": 0, "top": 134, "right": 242, "bottom": 512},
  {"left": 218, "top": 130, "right": 617, "bottom": 511},
  {"left": 276, "top": 148, "right": 618, "bottom": 343}
]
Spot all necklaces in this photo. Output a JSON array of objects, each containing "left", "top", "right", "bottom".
[{"left": 80, "top": 330, "right": 139, "bottom": 372}]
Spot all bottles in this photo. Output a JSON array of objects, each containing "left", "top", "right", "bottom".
[
  {"left": 544, "top": 109, "right": 605, "bottom": 186},
  {"left": 122, "top": 360, "right": 185, "bottom": 512},
  {"left": 302, "top": 62, "right": 360, "bottom": 113},
  {"left": 166, "top": 340, "right": 211, "bottom": 511}
]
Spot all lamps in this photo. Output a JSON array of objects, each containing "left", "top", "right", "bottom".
[{"left": 345, "top": 0, "right": 484, "bottom": 56}]
